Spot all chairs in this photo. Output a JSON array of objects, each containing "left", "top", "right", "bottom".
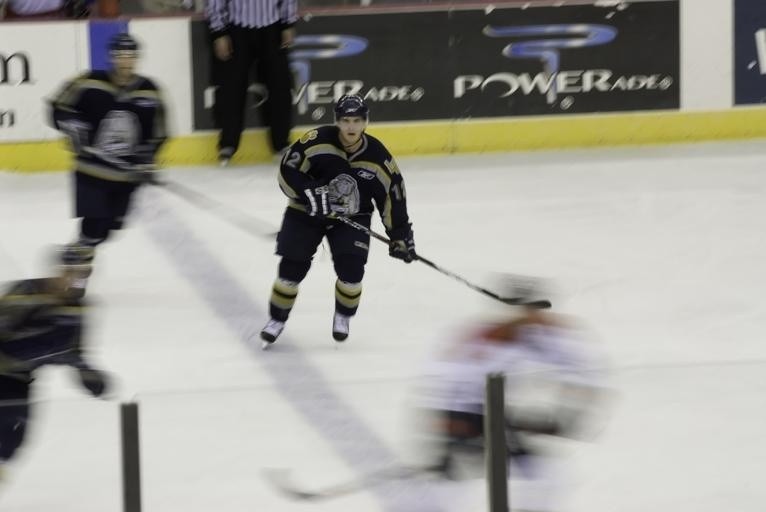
[
  {"left": 260, "top": 321, "right": 283, "bottom": 343},
  {"left": 219, "top": 135, "right": 236, "bottom": 158},
  {"left": 333, "top": 312, "right": 350, "bottom": 340}
]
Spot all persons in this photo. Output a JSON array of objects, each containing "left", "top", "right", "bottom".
[
  {"left": 203, "top": 0, "right": 300, "bottom": 160},
  {"left": 260, "top": 92, "right": 419, "bottom": 343},
  {"left": 53, "top": 37, "right": 169, "bottom": 299},
  {"left": 415, "top": 278, "right": 607, "bottom": 482},
  {"left": 0, "top": 245, "right": 122, "bottom": 476}
]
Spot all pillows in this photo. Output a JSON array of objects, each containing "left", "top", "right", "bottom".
[
  {"left": 333, "top": 93, "right": 371, "bottom": 127},
  {"left": 109, "top": 33, "right": 137, "bottom": 54}
]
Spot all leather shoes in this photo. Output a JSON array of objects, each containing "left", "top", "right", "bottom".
[
  {"left": 387, "top": 228, "right": 416, "bottom": 261},
  {"left": 308, "top": 187, "right": 348, "bottom": 220}
]
